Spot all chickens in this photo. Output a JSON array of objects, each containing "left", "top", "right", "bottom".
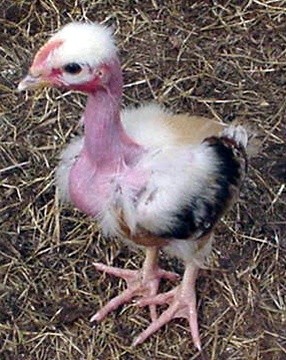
[{"left": 16, "top": 20, "right": 264, "bottom": 349}]
